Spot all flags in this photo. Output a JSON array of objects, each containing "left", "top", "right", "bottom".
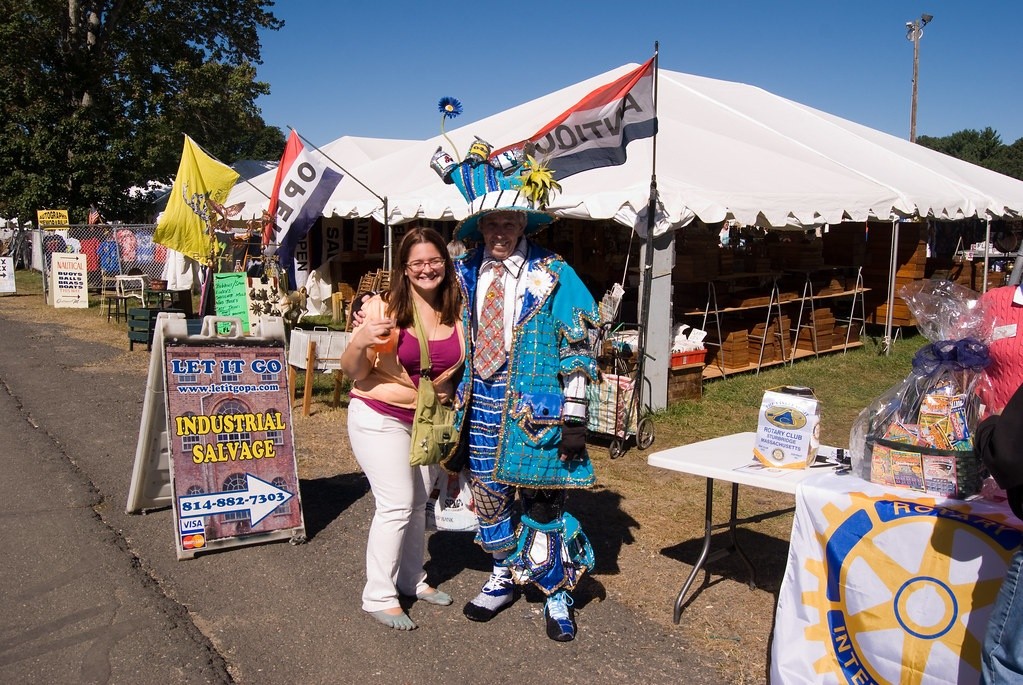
[
  {"left": 260, "top": 129, "right": 344, "bottom": 270},
  {"left": 152, "top": 134, "right": 241, "bottom": 267},
  {"left": 89, "top": 206, "right": 99, "bottom": 228},
  {"left": 486, "top": 57, "right": 657, "bottom": 182}
]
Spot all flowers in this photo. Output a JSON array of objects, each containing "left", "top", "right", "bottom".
[
  {"left": 523, "top": 269, "right": 555, "bottom": 302},
  {"left": 514, "top": 153, "right": 562, "bottom": 205},
  {"left": 438, "top": 96, "right": 463, "bottom": 163}
]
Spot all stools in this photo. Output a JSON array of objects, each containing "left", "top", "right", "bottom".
[{"left": 105, "top": 297, "right": 129, "bottom": 324}]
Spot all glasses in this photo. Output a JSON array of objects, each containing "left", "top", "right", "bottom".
[{"left": 404, "top": 258, "right": 446, "bottom": 273}]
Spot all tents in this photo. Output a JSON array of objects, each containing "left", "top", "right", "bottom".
[{"left": 152, "top": 62, "right": 1023, "bottom": 419}]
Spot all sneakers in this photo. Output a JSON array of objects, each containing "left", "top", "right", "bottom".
[
  {"left": 462, "top": 570, "right": 516, "bottom": 622},
  {"left": 542, "top": 590, "right": 576, "bottom": 641}
]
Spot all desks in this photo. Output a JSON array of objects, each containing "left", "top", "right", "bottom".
[
  {"left": 967, "top": 253, "right": 1017, "bottom": 262},
  {"left": 114, "top": 274, "right": 150, "bottom": 308},
  {"left": 647, "top": 432, "right": 1023, "bottom": 625},
  {"left": 143, "top": 289, "right": 176, "bottom": 310}
]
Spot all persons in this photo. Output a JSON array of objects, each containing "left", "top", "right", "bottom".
[
  {"left": 340, "top": 141, "right": 598, "bottom": 642},
  {"left": 945, "top": 271, "right": 1023, "bottom": 685}
]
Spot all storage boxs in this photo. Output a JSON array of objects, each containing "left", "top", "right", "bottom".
[{"left": 669, "top": 348, "right": 708, "bottom": 366}]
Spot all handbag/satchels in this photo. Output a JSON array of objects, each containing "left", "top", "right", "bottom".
[
  {"left": 423, "top": 468, "right": 481, "bottom": 532},
  {"left": 409, "top": 376, "right": 460, "bottom": 466}
]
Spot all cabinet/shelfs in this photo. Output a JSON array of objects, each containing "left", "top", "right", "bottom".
[
  {"left": 671, "top": 271, "right": 816, "bottom": 379},
  {"left": 784, "top": 264, "right": 872, "bottom": 369},
  {"left": 289, "top": 325, "right": 352, "bottom": 416}
]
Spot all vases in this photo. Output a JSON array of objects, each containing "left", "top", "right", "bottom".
[{"left": 150, "top": 280, "right": 168, "bottom": 291}]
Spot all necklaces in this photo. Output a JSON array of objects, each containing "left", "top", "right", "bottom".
[{"left": 411, "top": 296, "right": 440, "bottom": 368}]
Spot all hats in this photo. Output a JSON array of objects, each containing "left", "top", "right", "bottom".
[{"left": 430, "top": 96, "right": 558, "bottom": 238}]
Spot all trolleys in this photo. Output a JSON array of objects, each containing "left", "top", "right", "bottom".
[{"left": 580, "top": 322, "right": 655, "bottom": 459}]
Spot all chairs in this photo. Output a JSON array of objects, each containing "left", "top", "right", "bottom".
[
  {"left": 100, "top": 269, "right": 117, "bottom": 309},
  {"left": 98, "top": 276, "right": 117, "bottom": 317}
]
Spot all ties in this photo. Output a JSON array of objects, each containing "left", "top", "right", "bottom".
[{"left": 472, "top": 262, "right": 508, "bottom": 380}]
